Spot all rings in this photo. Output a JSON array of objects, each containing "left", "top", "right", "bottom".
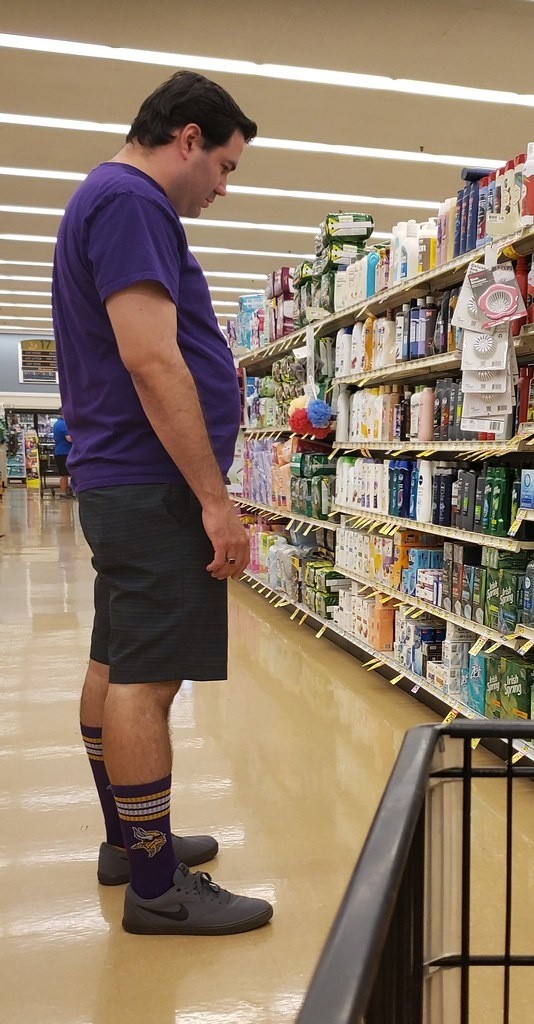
[{"left": 227, "top": 558, "right": 236, "bottom": 565}]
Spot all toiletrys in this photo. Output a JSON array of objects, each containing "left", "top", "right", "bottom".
[{"left": 334, "top": 141, "right": 533, "bottom": 538}]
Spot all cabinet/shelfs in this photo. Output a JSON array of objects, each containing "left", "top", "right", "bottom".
[{"left": 0, "top": 141, "right": 534, "bottom": 766}]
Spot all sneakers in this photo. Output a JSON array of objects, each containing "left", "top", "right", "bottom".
[
  {"left": 97, "top": 831, "right": 219, "bottom": 886},
  {"left": 121, "top": 863, "right": 273, "bottom": 935}
]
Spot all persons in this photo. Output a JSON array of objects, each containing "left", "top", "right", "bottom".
[
  {"left": 51, "top": 70, "right": 275, "bottom": 935},
  {"left": 53, "top": 407, "right": 76, "bottom": 501}
]
